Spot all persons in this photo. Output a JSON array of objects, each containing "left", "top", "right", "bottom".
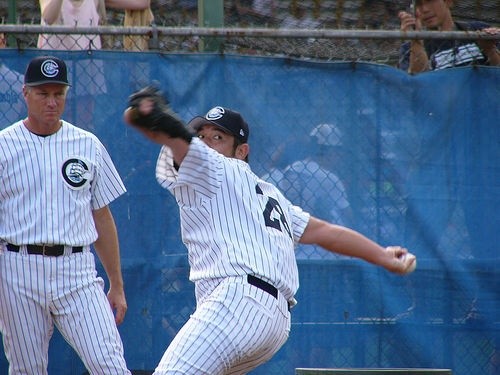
[
  {"left": 122, "top": 86, "right": 418, "bottom": 375},
  {"left": 236, "top": 0, "right": 500, "bottom": 374},
  {"left": 0, "top": 56, "right": 132, "bottom": 375},
  {"left": 0, "top": 0, "right": 151, "bottom": 134}
]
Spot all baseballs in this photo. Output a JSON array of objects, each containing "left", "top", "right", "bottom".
[{"left": 401, "top": 254, "right": 416, "bottom": 271}]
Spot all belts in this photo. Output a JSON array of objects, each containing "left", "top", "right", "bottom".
[
  {"left": 5, "top": 243, "right": 85, "bottom": 255},
  {"left": 248, "top": 275, "right": 291, "bottom": 312}
]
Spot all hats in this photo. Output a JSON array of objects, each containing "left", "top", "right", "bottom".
[
  {"left": 311, "top": 123, "right": 342, "bottom": 146},
  {"left": 22, "top": 56, "right": 70, "bottom": 88},
  {"left": 188, "top": 106, "right": 249, "bottom": 144}
]
